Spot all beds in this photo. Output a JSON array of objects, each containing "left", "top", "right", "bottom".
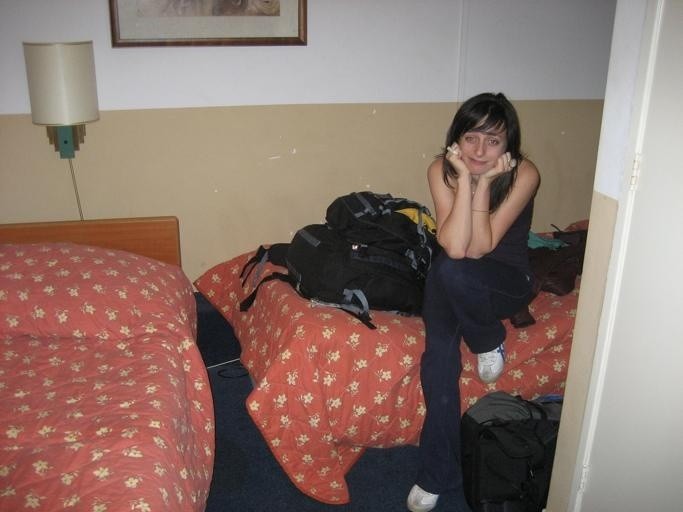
[
  {"left": 241, "top": 219, "right": 589, "bottom": 505},
  {"left": 0, "top": 216, "right": 216, "bottom": 512}
]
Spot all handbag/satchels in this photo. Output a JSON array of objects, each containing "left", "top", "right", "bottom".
[{"left": 460, "top": 390, "right": 564, "bottom": 511}]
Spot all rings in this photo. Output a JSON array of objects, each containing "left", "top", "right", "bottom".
[
  {"left": 446, "top": 146, "right": 453, "bottom": 153},
  {"left": 452, "top": 151, "right": 458, "bottom": 156}
]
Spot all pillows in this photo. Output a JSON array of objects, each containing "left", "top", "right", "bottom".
[{"left": 0, "top": 243, "right": 196, "bottom": 340}]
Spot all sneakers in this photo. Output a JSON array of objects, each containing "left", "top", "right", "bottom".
[
  {"left": 479, "top": 344, "right": 504, "bottom": 383},
  {"left": 406, "top": 482, "right": 439, "bottom": 511}
]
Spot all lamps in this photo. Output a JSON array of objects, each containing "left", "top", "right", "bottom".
[{"left": 22, "top": 39, "right": 101, "bottom": 161}]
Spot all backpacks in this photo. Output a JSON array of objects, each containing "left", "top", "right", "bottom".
[{"left": 286, "top": 191, "right": 437, "bottom": 314}]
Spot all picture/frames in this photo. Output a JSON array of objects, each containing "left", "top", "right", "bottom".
[{"left": 109, "top": 0, "right": 307, "bottom": 48}]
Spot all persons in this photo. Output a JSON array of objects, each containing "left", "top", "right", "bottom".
[{"left": 405, "top": 89, "right": 543, "bottom": 512}]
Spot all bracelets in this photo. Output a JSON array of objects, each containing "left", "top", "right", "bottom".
[{"left": 472, "top": 209, "right": 489, "bottom": 213}]
